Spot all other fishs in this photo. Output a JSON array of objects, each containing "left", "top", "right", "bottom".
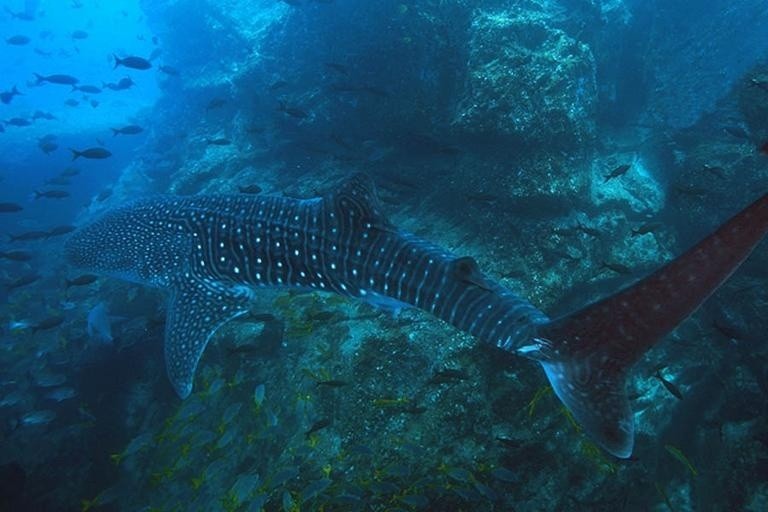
[{"left": 0, "top": 1, "right": 766, "bottom": 512}]
